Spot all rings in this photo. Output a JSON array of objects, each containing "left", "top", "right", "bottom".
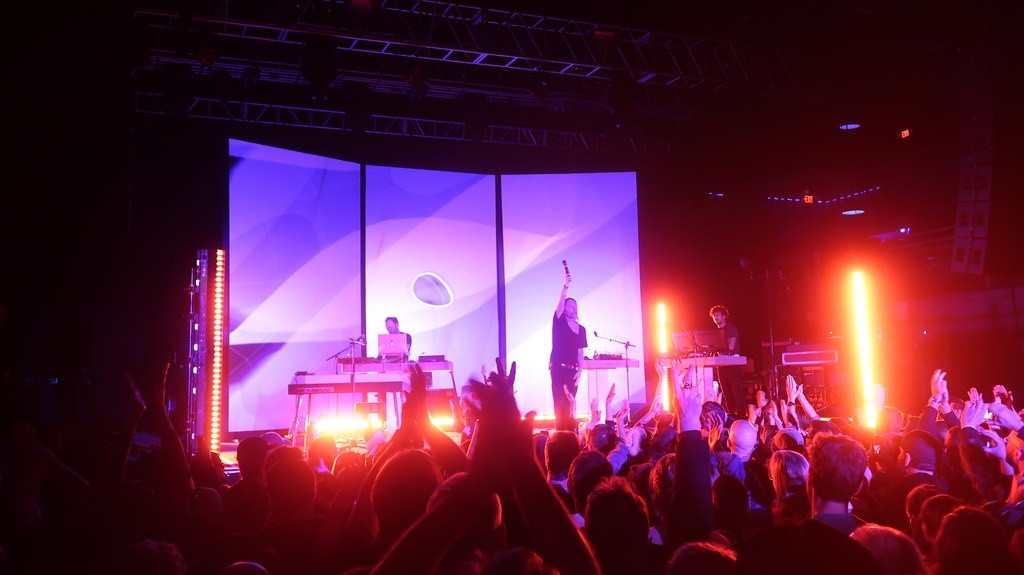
[
  {"left": 684, "top": 383, "right": 691, "bottom": 389},
  {"left": 973, "top": 403, "right": 976, "bottom": 406}
]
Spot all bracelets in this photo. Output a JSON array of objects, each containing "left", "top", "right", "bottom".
[
  {"left": 569, "top": 406, "right": 575, "bottom": 410},
  {"left": 788, "top": 402, "right": 795, "bottom": 406}
]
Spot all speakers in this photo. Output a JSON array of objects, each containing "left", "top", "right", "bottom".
[{"left": 774, "top": 365, "right": 828, "bottom": 426}]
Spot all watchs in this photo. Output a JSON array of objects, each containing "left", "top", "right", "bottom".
[{"left": 928, "top": 396, "right": 941, "bottom": 405}]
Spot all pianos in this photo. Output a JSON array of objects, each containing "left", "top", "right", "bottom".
[
  {"left": 659, "top": 354, "right": 750, "bottom": 369},
  {"left": 288, "top": 370, "right": 414, "bottom": 395},
  {"left": 337, "top": 354, "right": 454, "bottom": 373}
]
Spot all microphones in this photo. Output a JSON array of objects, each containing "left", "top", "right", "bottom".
[
  {"left": 594, "top": 332, "right": 597, "bottom": 336},
  {"left": 562, "top": 260, "right": 571, "bottom": 281},
  {"left": 350, "top": 334, "right": 365, "bottom": 345}
]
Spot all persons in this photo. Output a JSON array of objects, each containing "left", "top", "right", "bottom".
[
  {"left": 549, "top": 273, "right": 589, "bottom": 429},
  {"left": 709, "top": 305, "right": 747, "bottom": 416},
  {"left": 378, "top": 317, "right": 412, "bottom": 422},
  {"left": 0, "top": 357, "right": 1024, "bottom": 575}
]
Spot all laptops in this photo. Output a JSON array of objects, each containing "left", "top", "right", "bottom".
[
  {"left": 673, "top": 330, "right": 731, "bottom": 357},
  {"left": 378, "top": 334, "right": 409, "bottom": 357}
]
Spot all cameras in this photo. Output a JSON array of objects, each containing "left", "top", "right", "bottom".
[{"left": 983, "top": 410, "right": 995, "bottom": 421}]
